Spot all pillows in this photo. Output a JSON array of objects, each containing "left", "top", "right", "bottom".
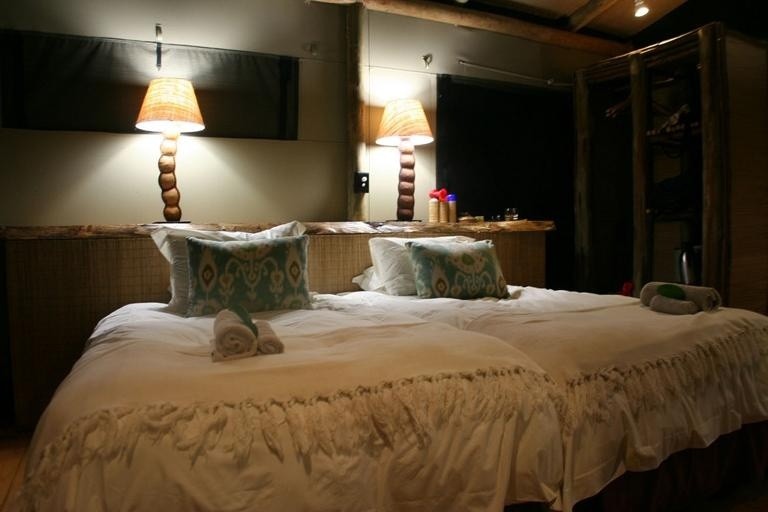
[
  {"left": 403, "top": 239, "right": 518, "bottom": 299},
  {"left": 368, "top": 232, "right": 478, "bottom": 296},
  {"left": 182, "top": 233, "right": 313, "bottom": 321},
  {"left": 349, "top": 265, "right": 388, "bottom": 296},
  {"left": 140, "top": 219, "right": 307, "bottom": 314}
]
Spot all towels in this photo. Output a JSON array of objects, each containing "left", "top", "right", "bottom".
[
  {"left": 639, "top": 278, "right": 723, "bottom": 308},
  {"left": 256, "top": 320, "right": 286, "bottom": 355},
  {"left": 208, "top": 302, "right": 258, "bottom": 364},
  {"left": 647, "top": 293, "right": 697, "bottom": 315}
]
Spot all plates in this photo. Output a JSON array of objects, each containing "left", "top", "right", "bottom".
[{"left": 459, "top": 217, "right": 476, "bottom": 224}]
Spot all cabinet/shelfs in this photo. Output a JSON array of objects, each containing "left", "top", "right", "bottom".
[{"left": 574, "top": 23, "right": 728, "bottom": 305}]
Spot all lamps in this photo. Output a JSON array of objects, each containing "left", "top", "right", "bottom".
[
  {"left": 376, "top": 98, "right": 436, "bottom": 222},
  {"left": 634, "top": 0, "right": 648, "bottom": 19},
  {"left": 133, "top": 74, "right": 208, "bottom": 225}
]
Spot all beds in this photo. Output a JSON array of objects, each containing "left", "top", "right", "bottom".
[
  {"left": 329, "top": 274, "right": 768, "bottom": 512},
  {"left": 23, "top": 296, "right": 559, "bottom": 512}
]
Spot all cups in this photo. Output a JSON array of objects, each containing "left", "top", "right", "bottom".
[
  {"left": 474, "top": 215, "right": 484, "bottom": 223},
  {"left": 504, "top": 207, "right": 518, "bottom": 221}
]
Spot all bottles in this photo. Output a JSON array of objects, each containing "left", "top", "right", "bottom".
[{"left": 428, "top": 194, "right": 457, "bottom": 224}]
